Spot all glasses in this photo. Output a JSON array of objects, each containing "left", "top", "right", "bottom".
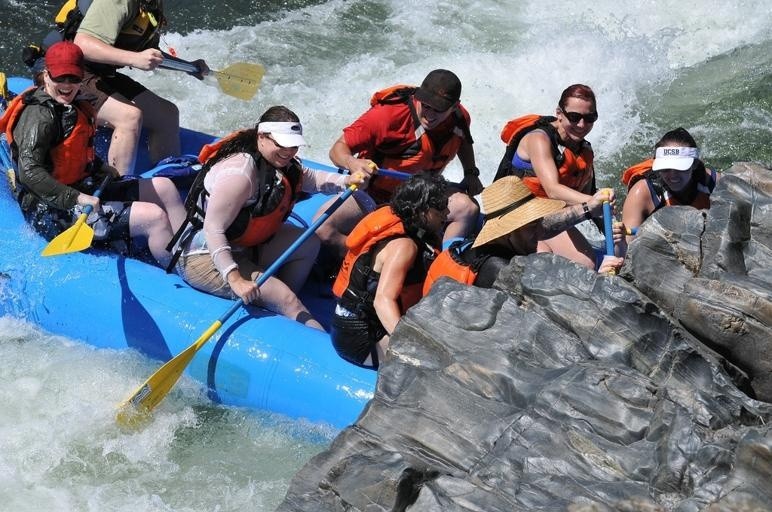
[
  {"left": 430, "top": 200, "right": 449, "bottom": 210},
  {"left": 562, "top": 107, "right": 598, "bottom": 123},
  {"left": 48, "top": 74, "right": 82, "bottom": 84}
]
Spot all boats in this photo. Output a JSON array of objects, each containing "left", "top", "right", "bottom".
[{"left": 0, "top": 77, "right": 620, "bottom": 431}]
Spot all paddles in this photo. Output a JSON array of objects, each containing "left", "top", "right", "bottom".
[
  {"left": 42, "top": 173, "right": 111, "bottom": 257},
  {"left": 118, "top": 174, "right": 365, "bottom": 435},
  {"left": 160, "top": 58, "right": 266, "bottom": 100}
]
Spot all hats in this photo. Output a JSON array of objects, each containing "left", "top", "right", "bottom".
[
  {"left": 471, "top": 175, "right": 567, "bottom": 248},
  {"left": 414, "top": 69, "right": 461, "bottom": 112},
  {"left": 258, "top": 120, "right": 307, "bottom": 148},
  {"left": 652, "top": 146, "right": 699, "bottom": 171},
  {"left": 45, "top": 42, "right": 86, "bottom": 79}
]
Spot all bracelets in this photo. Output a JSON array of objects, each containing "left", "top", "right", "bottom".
[
  {"left": 464, "top": 167, "right": 480, "bottom": 177},
  {"left": 581, "top": 202, "right": 592, "bottom": 220}
]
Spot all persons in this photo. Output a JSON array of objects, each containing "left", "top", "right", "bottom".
[
  {"left": 330, "top": 172, "right": 450, "bottom": 370},
  {"left": 0, "top": 41, "right": 192, "bottom": 273},
  {"left": 422, "top": 175, "right": 624, "bottom": 300},
  {"left": 310, "top": 68, "right": 484, "bottom": 259},
  {"left": 173, "top": 106, "right": 371, "bottom": 334},
  {"left": 612, "top": 127, "right": 721, "bottom": 259},
  {"left": 32, "top": 1, "right": 210, "bottom": 179},
  {"left": 493, "top": 84, "right": 618, "bottom": 270}
]
